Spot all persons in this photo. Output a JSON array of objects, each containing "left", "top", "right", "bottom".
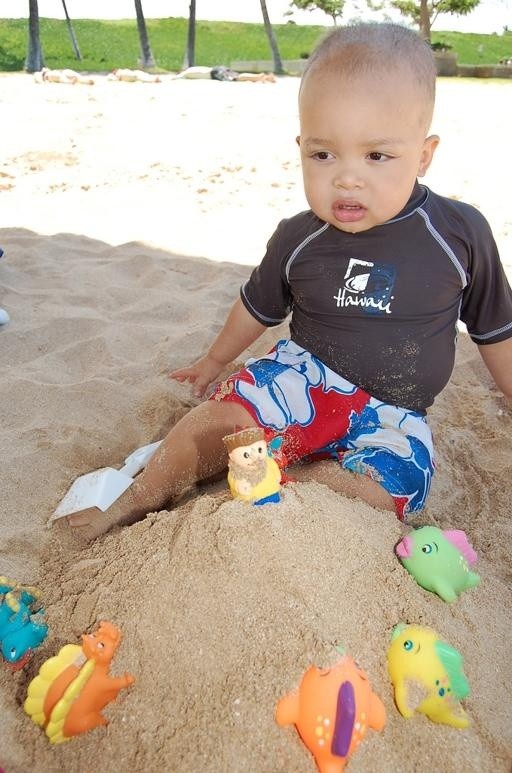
[{"left": 38, "top": 18, "right": 511, "bottom": 552}]
